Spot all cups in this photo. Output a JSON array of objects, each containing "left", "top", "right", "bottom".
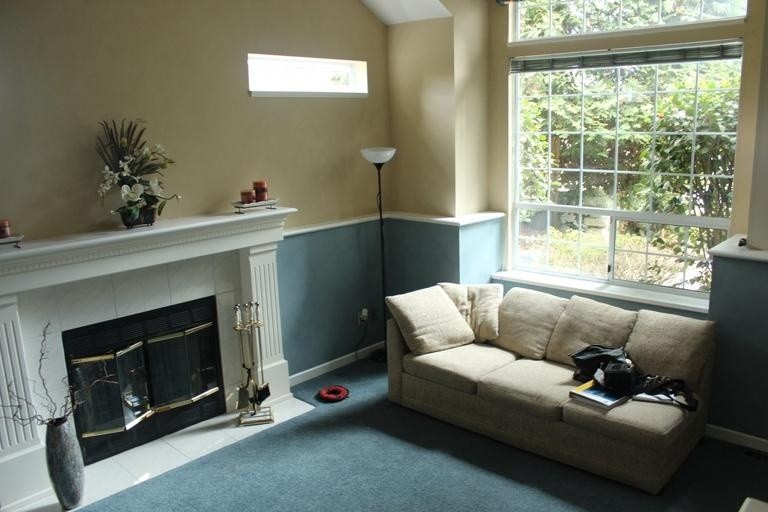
[{"left": 241, "top": 191, "right": 256, "bottom": 205}]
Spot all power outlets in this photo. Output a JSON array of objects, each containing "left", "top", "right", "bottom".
[{"left": 357, "top": 312, "right": 368, "bottom": 327}]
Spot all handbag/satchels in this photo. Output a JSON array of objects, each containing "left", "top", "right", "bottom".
[
  {"left": 570, "top": 344, "right": 625, "bottom": 383},
  {"left": 598, "top": 357, "right": 647, "bottom": 395}
]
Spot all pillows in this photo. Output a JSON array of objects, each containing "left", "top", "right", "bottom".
[
  {"left": 545, "top": 295, "right": 639, "bottom": 368},
  {"left": 437, "top": 283, "right": 504, "bottom": 341},
  {"left": 624, "top": 309, "right": 717, "bottom": 391},
  {"left": 385, "top": 284, "right": 475, "bottom": 356},
  {"left": 488, "top": 287, "right": 569, "bottom": 359}
]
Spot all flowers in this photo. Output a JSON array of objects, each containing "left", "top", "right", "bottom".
[{"left": 94, "top": 118, "right": 181, "bottom": 224}]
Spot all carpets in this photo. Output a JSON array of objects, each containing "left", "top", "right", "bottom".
[{"left": 73, "top": 348, "right": 768, "bottom": 512}]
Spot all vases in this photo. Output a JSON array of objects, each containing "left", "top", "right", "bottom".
[
  {"left": 45, "top": 417, "right": 85, "bottom": 510},
  {"left": 116, "top": 206, "right": 156, "bottom": 229}
]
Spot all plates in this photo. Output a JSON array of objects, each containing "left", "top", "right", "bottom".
[
  {"left": 0, "top": 234, "right": 24, "bottom": 245},
  {"left": 233, "top": 199, "right": 280, "bottom": 208}
]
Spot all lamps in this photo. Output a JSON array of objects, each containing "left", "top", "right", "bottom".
[{"left": 360, "top": 147, "right": 396, "bottom": 374}]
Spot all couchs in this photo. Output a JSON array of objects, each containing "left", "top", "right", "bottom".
[{"left": 385, "top": 283, "right": 720, "bottom": 497}]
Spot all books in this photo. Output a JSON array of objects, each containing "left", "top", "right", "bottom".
[
  {"left": 570, "top": 377, "right": 631, "bottom": 409},
  {"left": 633, "top": 392, "right": 674, "bottom": 404}
]
